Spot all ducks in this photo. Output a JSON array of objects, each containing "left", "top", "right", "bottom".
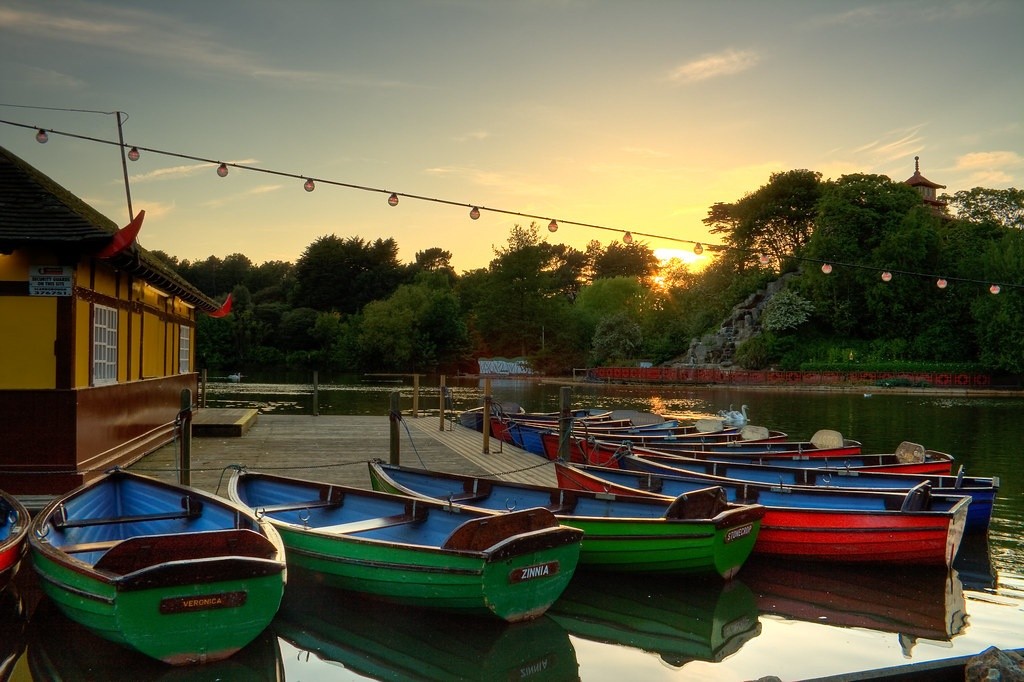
[
  {"left": 863, "top": 393, "right": 873, "bottom": 397},
  {"left": 716, "top": 403, "right": 752, "bottom": 421},
  {"left": 228, "top": 372, "right": 241, "bottom": 382}
]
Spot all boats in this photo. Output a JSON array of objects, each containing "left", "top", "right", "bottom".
[
  {"left": 0, "top": 488, "right": 32, "bottom": 592},
  {"left": 457, "top": 400, "right": 526, "bottom": 433},
  {"left": 368, "top": 455, "right": 765, "bottom": 582},
  {"left": 613, "top": 448, "right": 1001, "bottom": 537},
  {"left": 551, "top": 457, "right": 974, "bottom": 570},
  {"left": 0, "top": 554, "right": 974, "bottom": 682},
  {"left": 226, "top": 466, "right": 586, "bottom": 627},
  {"left": 20, "top": 464, "right": 288, "bottom": 669},
  {"left": 487, "top": 406, "right": 956, "bottom": 477}
]
